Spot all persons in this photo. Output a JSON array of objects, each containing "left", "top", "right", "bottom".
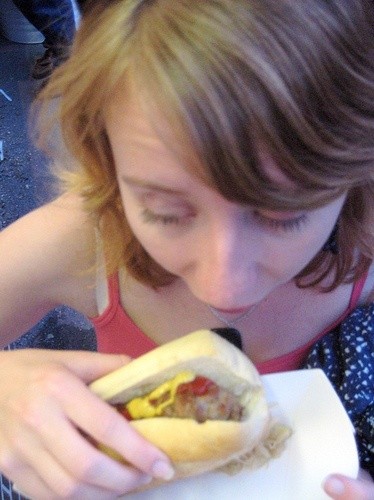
[
  {"left": 13, "top": 0, "right": 77, "bottom": 101},
  {"left": 0, "top": 0, "right": 374, "bottom": 500}
]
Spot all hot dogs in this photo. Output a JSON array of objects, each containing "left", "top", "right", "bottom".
[{"left": 80, "top": 327, "right": 265, "bottom": 488}]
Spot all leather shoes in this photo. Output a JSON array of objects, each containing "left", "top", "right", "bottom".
[{"left": 28, "top": 47, "right": 67, "bottom": 79}]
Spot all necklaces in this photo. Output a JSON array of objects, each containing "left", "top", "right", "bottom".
[{"left": 209, "top": 300, "right": 263, "bottom": 352}]
